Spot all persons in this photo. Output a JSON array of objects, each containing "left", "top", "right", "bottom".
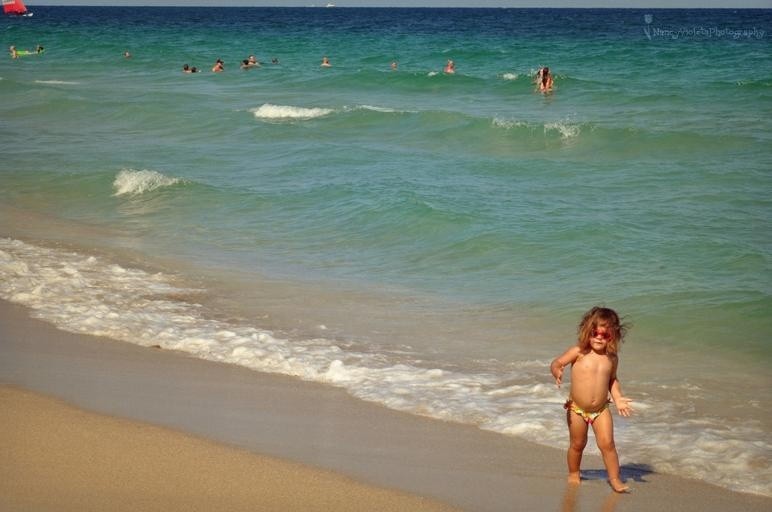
[
  {"left": 191, "top": 67, "right": 198, "bottom": 73},
  {"left": 537, "top": 69, "right": 544, "bottom": 80},
  {"left": 320, "top": 57, "right": 332, "bottom": 67},
  {"left": 212, "top": 59, "right": 225, "bottom": 72},
  {"left": 124, "top": 51, "right": 131, "bottom": 57},
  {"left": 391, "top": 62, "right": 398, "bottom": 71},
  {"left": 10, "top": 46, "right": 17, "bottom": 59},
  {"left": 248, "top": 56, "right": 260, "bottom": 66},
  {"left": 551, "top": 307, "right": 634, "bottom": 493},
  {"left": 240, "top": 60, "right": 255, "bottom": 70},
  {"left": 538, "top": 66, "right": 552, "bottom": 94},
  {"left": 443, "top": 61, "right": 455, "bottom": 76},
  {"left": 37, "top": 46, "right": 43, "bottom": 53},
  {"left": 272, "top": 58, "right": 280, "bottom": 65},
  {"left": 182, "top": 64, "right": 191, "bottom": 72}
]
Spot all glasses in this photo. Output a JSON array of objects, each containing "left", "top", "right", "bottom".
[{"left": 588, "top": 328, "right": 611, "bottom": 339}]
